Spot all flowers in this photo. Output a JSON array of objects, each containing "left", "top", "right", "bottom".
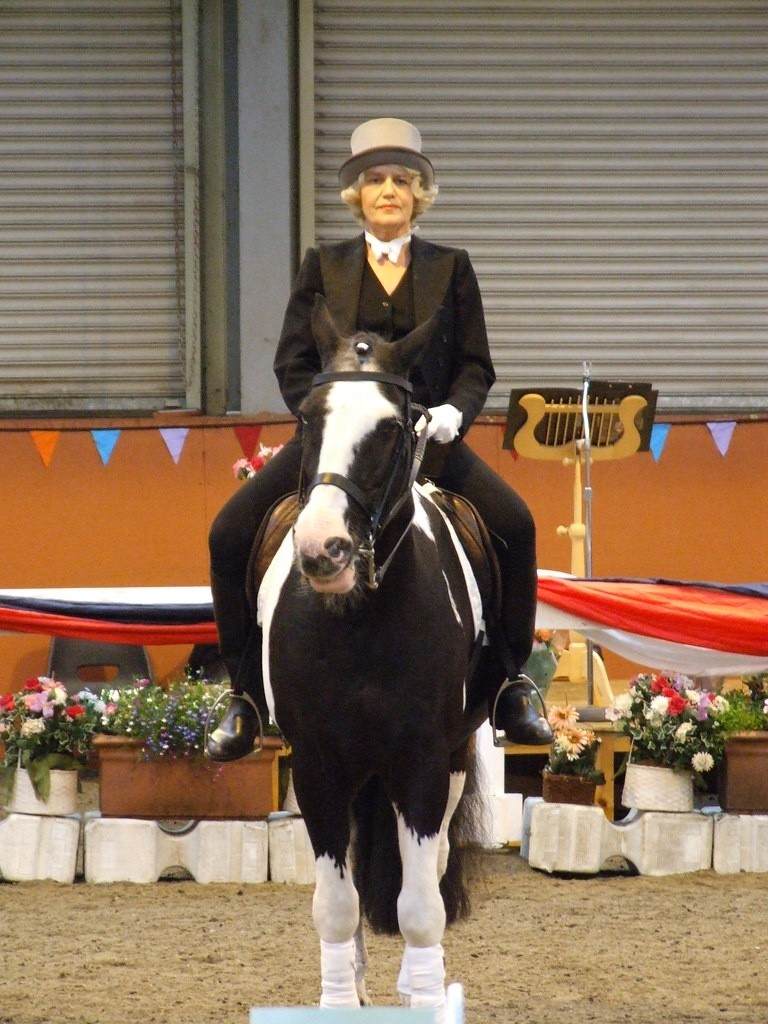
[
  {"left": 604, "top": 670, "right": 731, "bottom": 791},
  {"left": 544, "top": 704, "right": 607, "bottom": 786},
  {"left": 715, "top": 668, "right": 768, "bottom": 737},
  {"left": 93, "top": 663, "right": 284, "bottom": 783},
  {"left": 0, "top": 675, "right": 101, "bottom": 821}
]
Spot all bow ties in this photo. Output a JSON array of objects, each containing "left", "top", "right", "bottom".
[{"left": 364, "top": 226, "right": 421, "bottom": 263}]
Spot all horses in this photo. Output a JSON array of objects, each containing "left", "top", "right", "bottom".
[{"left": 241, "top": 289, "right": 513, "bottom": 1012}]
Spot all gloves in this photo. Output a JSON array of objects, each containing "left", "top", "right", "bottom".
[{"left": 415, "top": 404, "right": 462, "bottom": 443}]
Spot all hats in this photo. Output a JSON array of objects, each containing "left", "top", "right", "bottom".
[{"left": 338, "top": 118, "right": 435, "bottom": 189}]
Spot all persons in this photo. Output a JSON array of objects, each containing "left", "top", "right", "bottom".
[{"left": 203, "top": 117, "right": 555, "bottom": 763}]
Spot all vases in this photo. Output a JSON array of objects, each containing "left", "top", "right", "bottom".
[
  {"left": 541, "top": 768, "right": 597, "bottom": 805},
  {"left": 621, "top": 763, "right": 695, "bottom": 812},
  {"left": 93, "top": 732, "right": 284, "bottom": 821},
  {"left": 717, "top": 730, "right": 768, "bottom": 817},
  {"left": 0, "top": 762, "right": 80, "bottom": 815}
]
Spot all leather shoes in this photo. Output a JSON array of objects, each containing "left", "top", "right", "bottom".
[
  {"left": 209, "top": 703, "right": 269, "bottom": 762},
  {"left": 489, "top": 680, "right": 555, "bottom": 746}
]
